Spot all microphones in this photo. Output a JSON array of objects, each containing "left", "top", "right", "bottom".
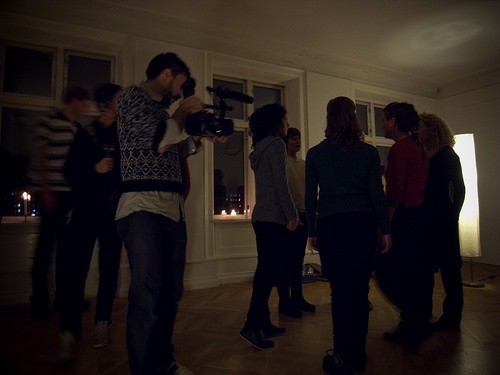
[{"left": 207, "top": 86, "right": 254, "bottom": 104}]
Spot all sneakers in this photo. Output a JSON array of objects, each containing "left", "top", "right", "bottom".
[
  {"left": 173, "top": 360, "right": 195, "bottom": 375},
  {"left": 58, "top": 332, "right": 76, "bottom": 361},
  {"left": 279, "top": 296, "right": 315, "bottom": 317},
  {"left": 239, "top": 327, "right": 275, "bottom": 351},
  {"left": 91, "top": 320, "right": 112, "bottom": 348},
  {"left": 261, "top": 324, "right": 289, "bottom": 336}
]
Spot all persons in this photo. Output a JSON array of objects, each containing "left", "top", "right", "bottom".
[
  {"left": 303, "top": 95, "right": 394, "bottom": 375},
  {"left": 54, "top": 81, "right": 122, "bottom": 360},
  {"left": 275, "top": 127, "right": 317, "bottom": 317},
  {"left": 115, "top": 50, "right": 232, "bottom": 375},
  {"left": 28, "top": 86, "right": 96, "bottom": 322},
  {"left": 240, "top": 104, "right": 303, "bottom": 352},
  {"left": 214, "top": 166, "right": 230, "bottom": 216},
  {"left": 413, "top": 112, "right": 466, "bottom": 328},
  {"left": 375, "top": 101, "right": 430, "bottom": 344}
]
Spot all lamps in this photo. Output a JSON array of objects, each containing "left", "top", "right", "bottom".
[{"left": 451, "top": 133, "right": 484, "bottom": 288}]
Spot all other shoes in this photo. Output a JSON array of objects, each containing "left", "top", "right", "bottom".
[
  {"left": 323, "top": 352, "right": 367, "bottom": 375},
  {"left": 53, "top": 298, "right": 91, "bottom": 311},
  {"left": 383, "top": 321, "right": 432, "bottom": 343},
  {"left": 431, "top": 316, "right": 460, "bottom": 330}
]
